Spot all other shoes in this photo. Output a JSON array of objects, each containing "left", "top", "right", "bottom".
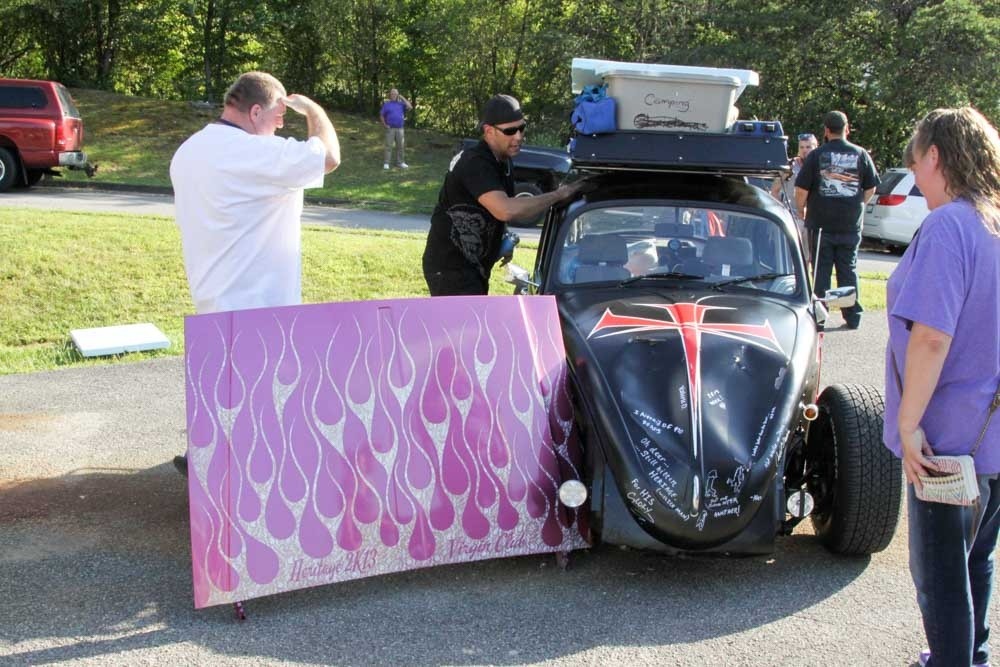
[{"left": 846, "top": 322, "right": 859, "bottom": 330}]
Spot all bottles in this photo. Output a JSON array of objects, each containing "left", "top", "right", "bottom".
[{"left": 498, "top": 232, "right": 520, "bottom": 257}]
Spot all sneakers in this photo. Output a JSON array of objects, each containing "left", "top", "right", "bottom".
[
  {"left": 397, "top": 162, "right": 409, "bottom": 169},
  {"left": 917, "top": 648, "right": 990, "bottom": 667},
  {"left": 384, "top": 163, "right": 390, "bottom": 170}
]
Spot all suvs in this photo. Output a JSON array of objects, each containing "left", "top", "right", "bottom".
[{"left": 0, "top": 78, "right": 98, "bottom": 194}]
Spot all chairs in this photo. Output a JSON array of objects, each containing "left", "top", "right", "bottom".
[
  {"left": 701, "top": 235, "right": 756, "bottom": 289},
  {"left": 574, "top": 234, "right": 630, "bottom": 284}
]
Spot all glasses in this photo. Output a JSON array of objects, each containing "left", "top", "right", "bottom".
[
  {"left": 491, "top": 120, "right": 527, "bottom": 136},
  {"left": 797, "top": 133, "right": 815, "bottom": 141}
]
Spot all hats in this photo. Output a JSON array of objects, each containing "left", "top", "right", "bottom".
[{"left": 477, "top": 93, "right": 523, "bottom": 137}]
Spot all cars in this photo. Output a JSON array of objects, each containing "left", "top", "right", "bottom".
[
  {"left": 858, "top": 167, "right": 930, "bottom": 257},
  {"left": 496, "top": 117, "right": 904, "bottom": 560}
]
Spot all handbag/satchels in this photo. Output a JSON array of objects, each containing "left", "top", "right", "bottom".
[{"left": 913, "top": 454, "right": 982, "bottom": 506}]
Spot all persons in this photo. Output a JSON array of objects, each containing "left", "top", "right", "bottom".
[
  {"left": 422, "top": 93, "right": 588, "bottom": 297},
  {"left": 882, "top": 106, "right": 1000, "bottom": 667},
  {"left": 169, "top": 70, "right": 341, "bottom": 479},
  {"left": 380, "top": 89, "right": 413, "bottom": 169},
  {"left": 770, "top": 111, "right": 882, "bottom": 332}
]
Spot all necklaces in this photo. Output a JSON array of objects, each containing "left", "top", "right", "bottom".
[{"left": 217, "top": 116, "right": 242, "bottom": 130}]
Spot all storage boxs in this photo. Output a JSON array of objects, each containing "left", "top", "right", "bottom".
[{"left": 602, "top": 70, "right": 741, "bottom": 133}]
